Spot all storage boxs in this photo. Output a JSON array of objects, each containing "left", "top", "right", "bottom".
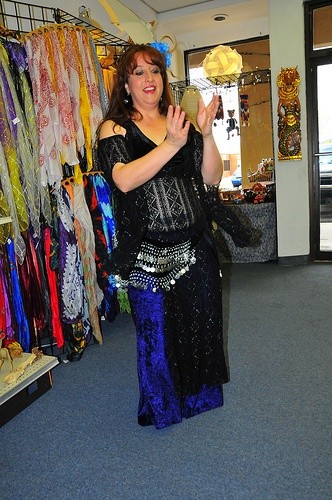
[{"left": 1, "top": 372, "right": 54, "bottom": 428}]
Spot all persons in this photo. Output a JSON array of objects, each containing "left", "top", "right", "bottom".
[{"left": 97, "top": 44, "right": 230, "bottom": 430}]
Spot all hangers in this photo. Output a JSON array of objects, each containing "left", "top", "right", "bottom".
[{"left": 2, "top": 5, "right": 108, "bottom": 198}]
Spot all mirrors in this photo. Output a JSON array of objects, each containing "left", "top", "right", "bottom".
[{"left": 185, "top": 36, "right": 276, "bottom": 192}]
